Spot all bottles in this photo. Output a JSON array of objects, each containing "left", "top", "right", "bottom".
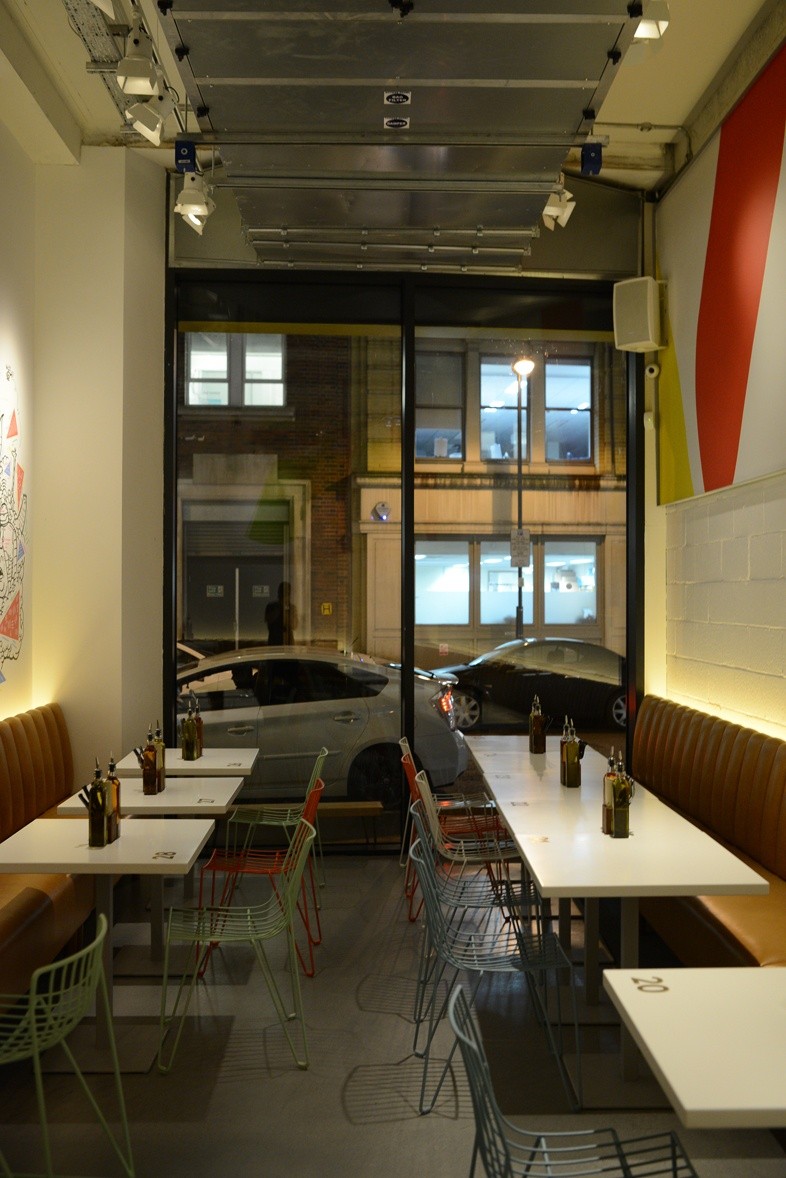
[
  {"left": 87, "top": 755, "right": 108, "bottom": 847},
  {"left": 155, "top": 720, "right": 166, "bottom": 793},
  {"left": 142, "top": 721, "right": 158, "bottom": 796},
  {"left": 532, "top": 697, "right": 546, "bottom": 754},
  {"left": 602, "top": 746, "right": 617, "bottom": 834},
  {"left": 565, "top": 720, "right": 581, "bottom": 788},
  {"left": 560, "top": 716, "right": 572, "bottom": 785},
  {"left": 104, "top": 750, "right": 121, "bottom": 845},
  {"left": 612, "top": 751, "right": 630, "bottom": 838},
  {"left": 193, "top": 699, "right": 203, "bottom": 758},
  {"left": 528, "top": 694, "right": 536, "bottom": 753},
  {"left": 184, "top": 700, "right": 197, "bottom": 761}
]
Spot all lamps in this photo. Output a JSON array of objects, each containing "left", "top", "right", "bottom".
[
  {"left": 116, "top": 6, "right": 166, "bottom": 98},
  {"left": 541, "top": 189, "right": 579, "bottom": 233},
  {"left": 633, "top": 0, "right": 671, "bottom": 41},
  {"left": 181, "top": 196, "right": 217, "bottom": 237},
  {"left": 173, "top": 171, "right": 209, "bottom": 216},
  {"left": 124, "top": 88, "right": 179, "bottom": 147}
]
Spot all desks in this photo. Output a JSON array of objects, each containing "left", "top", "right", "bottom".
[
  {"left": 600, "top": 966, "right": 786, "bottom": 1129},
  {"left": 463, "top": 734, "right": 772, "bottom": 1113},
  {"left": 108, "top": 746, "right": 262, "bottom": 909},
  {"left": 0, "top": 816, "right": 217, "bottom": 1075},
  {"left": 52, "top": 775, "right": 248, "bottom": 976}
]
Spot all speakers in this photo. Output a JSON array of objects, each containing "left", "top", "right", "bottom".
[{"left": 613, "top": 275, "right": 667, "bottom": 353}]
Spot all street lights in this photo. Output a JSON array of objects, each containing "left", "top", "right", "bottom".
[{"left": 510, "top": 352, "right": 535, "bottom": 639}]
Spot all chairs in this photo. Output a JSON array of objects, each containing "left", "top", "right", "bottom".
[
  {"left": 154, "top": 817, "right": 319, "bottom": 1072},
  {"left": 220, "top": 744, "right": 331, "bottom": 915},
  {"left": 193, "top": 774, "right": 328, "bottom": 984},
  {"left": 396, "top": 735, "right": 589, "bottom": 1119},
  {"left": 0, "top": 912, "right": 137, "bottom": 1178},
  {"left": 446, "top": 984, "right": 704, "bottom": 1178}
]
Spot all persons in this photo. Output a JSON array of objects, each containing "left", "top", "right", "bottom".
[
  {"left": 264, "top": 582, "right": 298, "bottom": 645},
  {"left": 227, "top": 665, "right": 259, "bottom": 707}
]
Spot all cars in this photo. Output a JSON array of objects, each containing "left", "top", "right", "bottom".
[
  {"left": 175, "top": 640, "right": 259, "bottom": 696},
  {"left": 431, "top": 636, "right": 630, "bottom": 734},
  {"left": 174, "top": 642, "right": 469, "bottom": 804}
]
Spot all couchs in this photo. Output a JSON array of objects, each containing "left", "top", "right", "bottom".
[
  {"left": 0, "top": 701, "right": 121, "bottom": 1028},
  {"left": 631, "top": 693, "right": 786, "bottom": 967}
]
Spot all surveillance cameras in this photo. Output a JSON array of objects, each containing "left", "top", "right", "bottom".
[{"left": 645, "top": 363, "right": 660, "bottom": 378}]
